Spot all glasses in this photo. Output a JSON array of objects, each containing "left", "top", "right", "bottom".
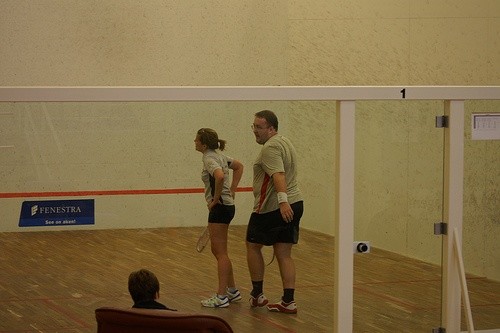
[{"left": 251, "top": 124, "right": 271, "bottom": 131}]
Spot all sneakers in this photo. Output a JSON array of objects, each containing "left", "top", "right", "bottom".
[
  {"left": 226, "top": 290, "right": 242, "bottom": 302},
  {"left": 248, "top": 294, "right": 268, "bottom": 307},
  {"left": 266, "top": 299, "right": 298, "bottom": 315},
  {"left": 200, "top": 293, "right": 230, "bottom": 308}
]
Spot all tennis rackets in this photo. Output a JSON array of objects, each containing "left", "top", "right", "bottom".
[
  {"left": 196, "top": 226, "right": 210, "bottom": 252},
  {"left": 262, "top": 245, "right": 275, "bottom": 266}
]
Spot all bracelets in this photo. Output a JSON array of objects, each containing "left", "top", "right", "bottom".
[{"left": 277, "top": 192, "right": 288, "bottom": 204}]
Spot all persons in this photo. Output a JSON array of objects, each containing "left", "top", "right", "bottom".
[
  {"left": 245, "top": 110, "right": 304, "bottom": 314},
  {"left": 128, "top": 269, "right": 179, "bottom": 312},
  {"left": 194, "top": 128, "right": 243, "bottom": 307}
]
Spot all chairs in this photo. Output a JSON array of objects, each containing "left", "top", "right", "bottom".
[{"left": 96, "top": 306, "right": 235, "bottom": 333}]
